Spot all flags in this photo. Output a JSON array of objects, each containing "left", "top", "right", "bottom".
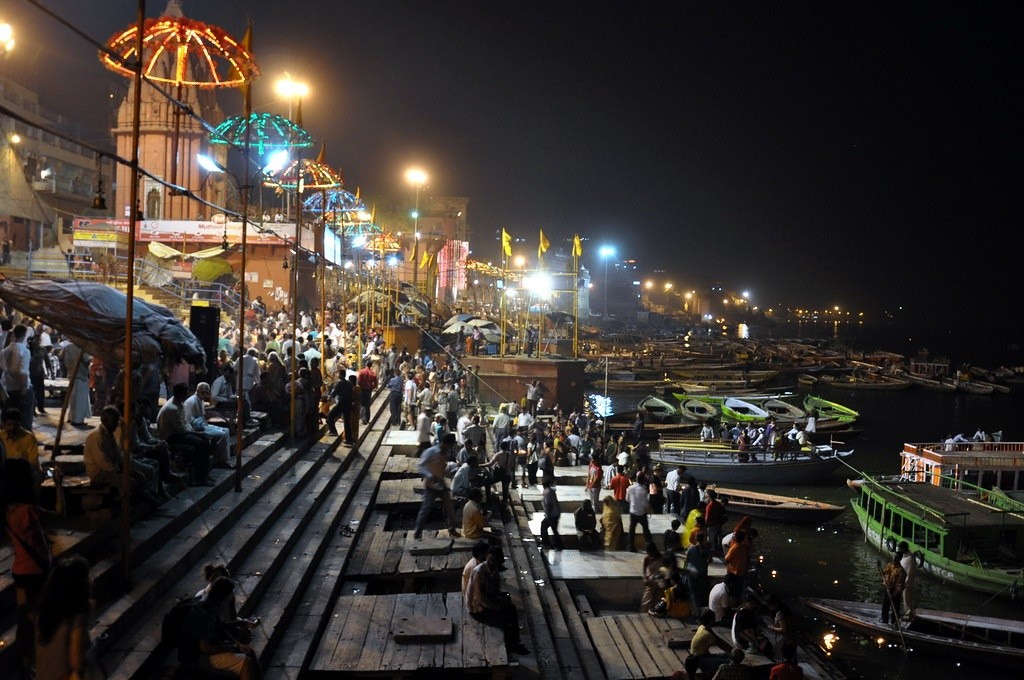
[
  {"left": 572, "top": 233, "right": 582, "bottom": 257},
  {"left": 538, "top": 228, "right": 551, "bottom": 258},
  {"left": 502, "top": 227, "right": 513, "bottom": 257}
]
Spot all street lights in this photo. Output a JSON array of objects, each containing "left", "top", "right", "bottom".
[
  {"left": 600, "top": 246, "right": 615, "bottom": 313},
  {"left": 406, "top": 169, "right": 428, "bottom": 287}
]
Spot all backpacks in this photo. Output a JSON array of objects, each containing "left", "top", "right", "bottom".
[{"left": 177, "top": 597, "right": 207, "bottom": 665}]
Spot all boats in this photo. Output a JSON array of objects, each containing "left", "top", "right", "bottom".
[
  {"left": 761, "top": 398, "right": 805, "bottom": 420},
  {"left": 911, "top": 352, "right": 1024, "bottom": 394},
  {"left": 597, "top": 419, "right": 701, "bottom": 435},
  {"left": 775, "top": 339, "right": 910, "bottom": 387},
  {"left": 680, "top": 397, "right": 718, "bottom": 421},
  {"left": 674, "top": 344, "right": 777, "bottom": 386},
  {"left": 637, "top": 394, "right": 678, "bottom": 423},
  {"left": 847, "top": 474, "right": 1024, "bottom": 596},
  {"left": 900, "top": 442, "right": 1024, "bottom": 508},
  {"left": 581, "top": 349, "right": 672, "bottom": 393},
  {"left": 694, "top": 486, "right": 841, "bottom": 526},
  {"left": 647, "top": 433, "right": 854, "bottom": 481},
  {"left": 720, "top": 396, "right": 769, "bottom": 421},
  {"left": 802, "top": 595, "right": 1024, "bottom": 665},
  {"left": 804, "top": 394, "right": 859, "bottom": 424}
]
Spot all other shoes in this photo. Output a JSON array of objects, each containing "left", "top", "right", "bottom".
[
  {"left": 246, "top": 419, "right": 260, "bottom": 425},
  {"left": 198, "top": 479, "right": 214, "bottom": 486},
  {"left": 71, "top": 422, "right": 87, "bottom": 426},
  {"left": 448, "top": 527, "right": 461, "bottom": 537},
  {"left": 342, "top": 441, "right": 354, "bottom": 446},
  {"left": 327, "top": 432, "right": 338, "bottom": 436},
  {"left": 414, "top": 533, "right": 422, "bottom": 541}
]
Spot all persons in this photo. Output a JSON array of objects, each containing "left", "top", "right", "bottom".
[
  {"left": 275, "top": 211, "right": 283, "bottom": 223},
  {"left": 197, "top": 211, "right": 203, "bottom": 220},
  {"left": 262, "top": 211, "right": 271, "bottom": 222},
  {"left": 0, "top": 237, "right": 986, "bottom": 680},
  {"left": 877, "top": 551, "right": 907, "bottom": 628},
  {"left": 897, "top": 541, "right": 915, "bottom": 620}
]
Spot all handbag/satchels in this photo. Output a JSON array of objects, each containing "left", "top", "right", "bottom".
[
  {"left": 493, "top": 466, "right": 506, "bottom": 480},
  {"left": 539, "top": 455, "right": 554, "bottom": 473}
]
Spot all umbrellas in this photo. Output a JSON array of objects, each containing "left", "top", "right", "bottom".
[{"left": 0, "top": 276, "right": 207, "bottom": 463}]
